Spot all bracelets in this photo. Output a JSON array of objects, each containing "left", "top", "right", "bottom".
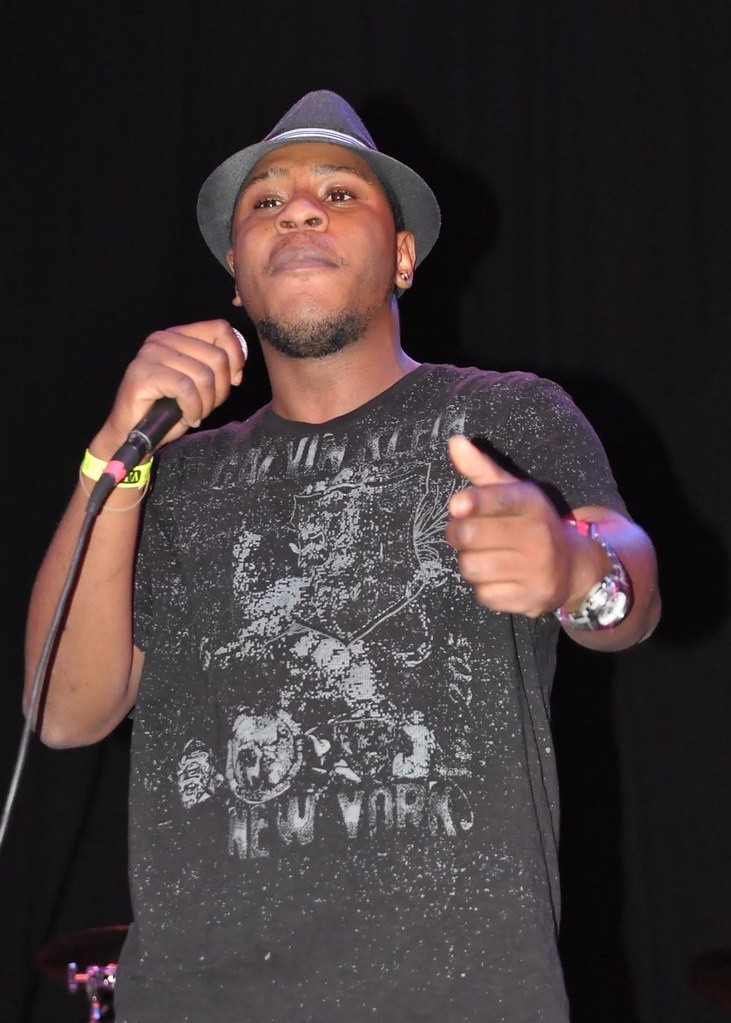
[
  {"left": 81, "top": 449, "right": 153, "bottom": 488},
  {"left": 79, "top": 470, "right": 150, "bottom": 512}
]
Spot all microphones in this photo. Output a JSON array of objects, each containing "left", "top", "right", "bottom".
[{"left": 86, "top": 327, "right": 248, "bottom": 514}]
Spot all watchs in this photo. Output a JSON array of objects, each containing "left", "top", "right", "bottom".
[{"left": 554, "top": 521, "right": 629, "bottom": 632}]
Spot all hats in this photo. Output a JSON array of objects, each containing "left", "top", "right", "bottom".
[{"left": 197, "top": 89, "right": 441, "bottom": 300}]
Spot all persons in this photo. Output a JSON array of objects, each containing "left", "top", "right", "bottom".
[{"left": 22, "top": 88, "right": 660, "bottom": 1023}]
